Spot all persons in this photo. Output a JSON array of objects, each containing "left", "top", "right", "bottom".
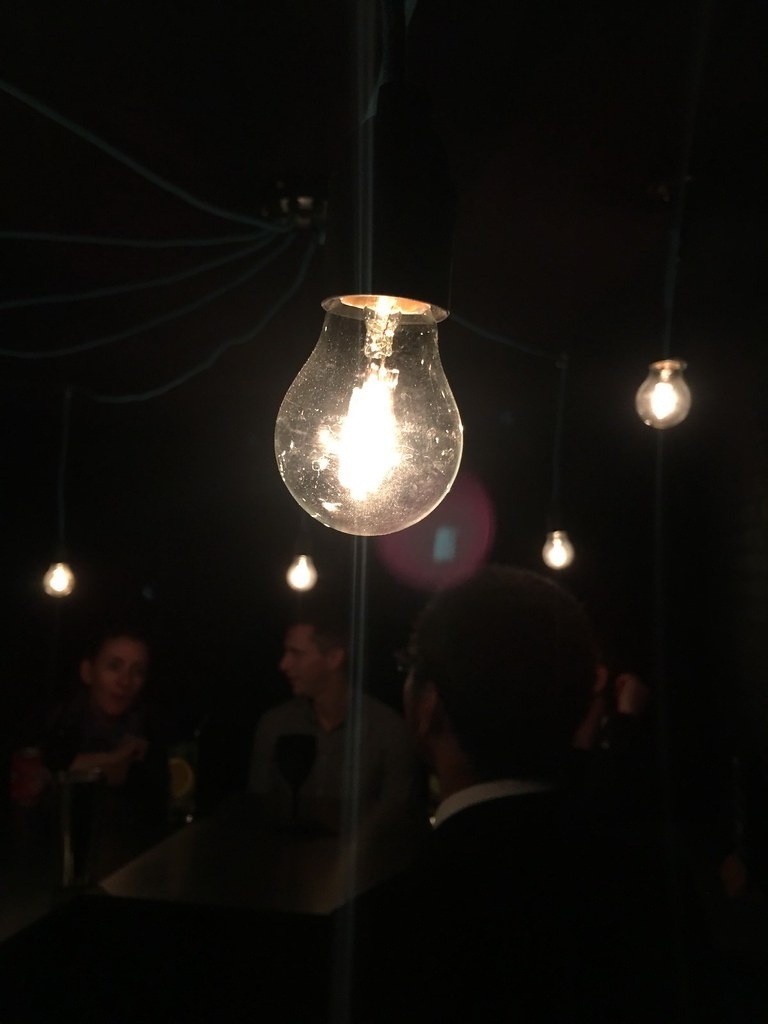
[
  {"left": 9, "top": 626, "right": 193, "bottom": 903},
  {"left": 243, "top": 614, "right": 420, "bottom": 834},
  {"left": 567, "top": 663, "right": 650, "bottom": 778},
  {"left": 247, "top": 568, "right": 740, "bottom": 1022}
]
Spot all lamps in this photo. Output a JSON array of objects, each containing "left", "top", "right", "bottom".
[
  {"left": 285, "top": 505, "right": 317, "bottom": 602},
  {"left": 630, "top": 4, "right": 731, "bottom": 437},
  {"left": 540, "top": 356, "right": 582, "bottom": 572},
  {"left": 38, "top": 382, "right": 87, "bottom": 605},
  {"left": 274, "top": 0, "right": 475, "bottom": 550}
]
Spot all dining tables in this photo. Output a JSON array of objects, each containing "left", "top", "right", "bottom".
[{"left": 85, "top": 793, "right": 429, "bottom": 1016}]
